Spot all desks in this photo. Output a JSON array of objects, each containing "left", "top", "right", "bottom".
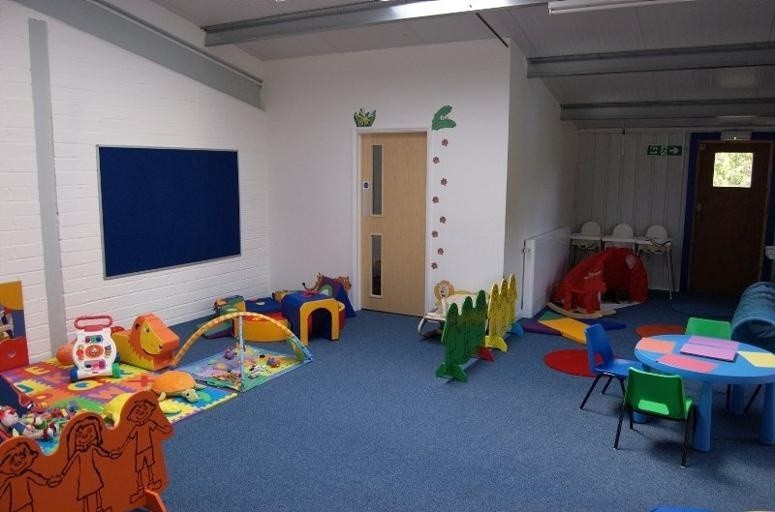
[{"left": 632, "top": 334, "right": 775, "bottom": 455}]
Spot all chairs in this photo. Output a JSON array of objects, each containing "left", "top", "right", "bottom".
[
  {"left": 610, "top": 365, "right": 701, "bottom": 470},
  {"left": 579, "top": 323, "right": 647, "bottom": 417},
  {"left": 414, "top": 290, "right": 488, "bottom": 347},
  {"left": 568, "top": 219, "right": 603, "bottom": 265},
  {"left": 684, "top": 316, "right": 735, "bottom": 411},
  {"left": 602, "top": 222, "right": 637, "bottom": 254},
  {"left": 636, "top": 224, "right": 677, "bottom": 301}
]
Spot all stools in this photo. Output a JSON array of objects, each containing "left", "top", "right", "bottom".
[{"left": 744, "top": 381, "right": 764, "bottom": 414}]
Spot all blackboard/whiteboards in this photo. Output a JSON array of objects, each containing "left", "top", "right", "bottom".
[{"left": 95, "top": 144, "right": 242, "bottom": 280}]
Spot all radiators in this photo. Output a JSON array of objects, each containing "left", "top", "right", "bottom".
[{"left": 522, "top": 224, "right": 572, "bottom": 320}]
